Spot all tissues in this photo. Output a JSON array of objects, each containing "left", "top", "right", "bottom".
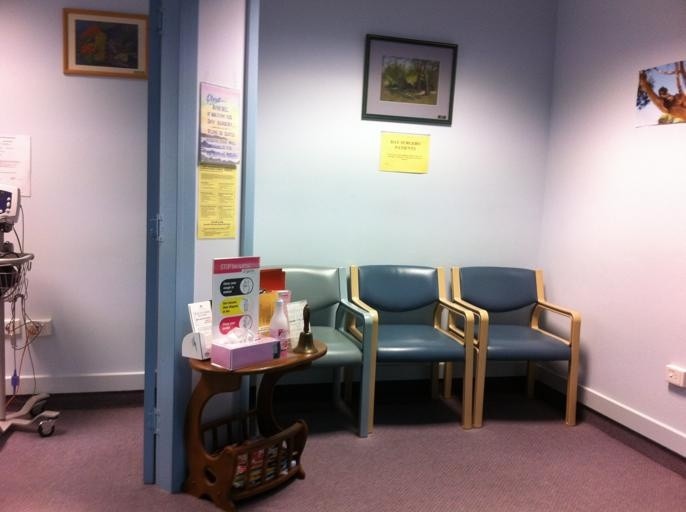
[{"left": 210, "top": 327, "right": 280, "bottom": 370}]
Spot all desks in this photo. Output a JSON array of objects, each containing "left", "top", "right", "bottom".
[{"left": 178, "top": 335, "right": 329, "bottom": 511}]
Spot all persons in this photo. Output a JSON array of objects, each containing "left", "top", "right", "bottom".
[{"left": 638, "top": 72, "right": 686, "bottom": 123}]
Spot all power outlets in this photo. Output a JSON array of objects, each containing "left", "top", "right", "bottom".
[
  {"left": 22, "top": 318, "right": 54, "bottom": 337},
  {"left": 2, "top": 318, "right": 21, "bottom": 337}
]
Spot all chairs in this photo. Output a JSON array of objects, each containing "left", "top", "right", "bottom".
[
  {"left": 339, "top": 259, "right": 477, "bottom": 437},
  {"left": 441, "top": 263, "right": 585, "bottom": 428},
  {"left": 254, "top": 262, "right": 375, "bottom": 443}
]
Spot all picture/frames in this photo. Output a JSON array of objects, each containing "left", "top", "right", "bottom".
[
  {"left": 359, "top": 34, "right": 460, "bottom": 127},
  {"left": 58, "top": 7, "right": 151, "bottom": 81}
]
